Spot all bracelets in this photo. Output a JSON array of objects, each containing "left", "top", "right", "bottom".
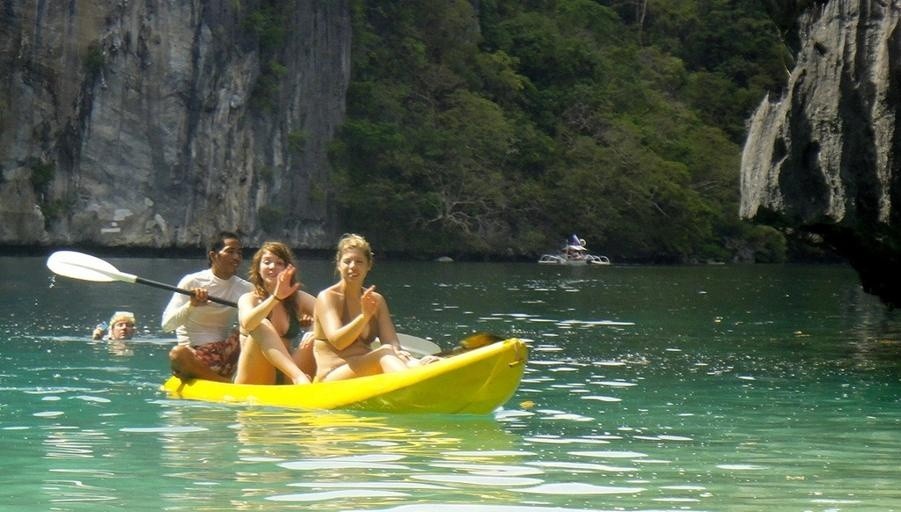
[{"left": 272, "top": 293, "right": 283, "bottom": 302}]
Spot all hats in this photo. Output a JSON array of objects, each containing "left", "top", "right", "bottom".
[
  {"left": 110, "top": 311, "right": 135, "bottom": 327},
  {"left": 579, "top": 237, "right": 586, "bottom": 246}
]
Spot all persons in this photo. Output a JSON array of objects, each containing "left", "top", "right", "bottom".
[
  {"left": 231, "top": 240, "right": 318, "bottom": 385},
  {"left": 92, "top": 309, "right": 136, "bottom": 341},
  {"left": 160, "top": 230, "right": 257, "bottom": 382},
  {"left": 311, "top": 232, "right": 444, "bottom": 382}
]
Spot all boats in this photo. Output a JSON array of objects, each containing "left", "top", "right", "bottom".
[
  {"left": 161, "top": 336, "right": 529, "bottom": 414},
  {"left": 538, "top": 234, "right": 609, "bottom": 266}
]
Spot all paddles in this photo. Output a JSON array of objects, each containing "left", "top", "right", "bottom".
[{"left": 46, "top": 250, "right": 442, "bottom": 358}]
